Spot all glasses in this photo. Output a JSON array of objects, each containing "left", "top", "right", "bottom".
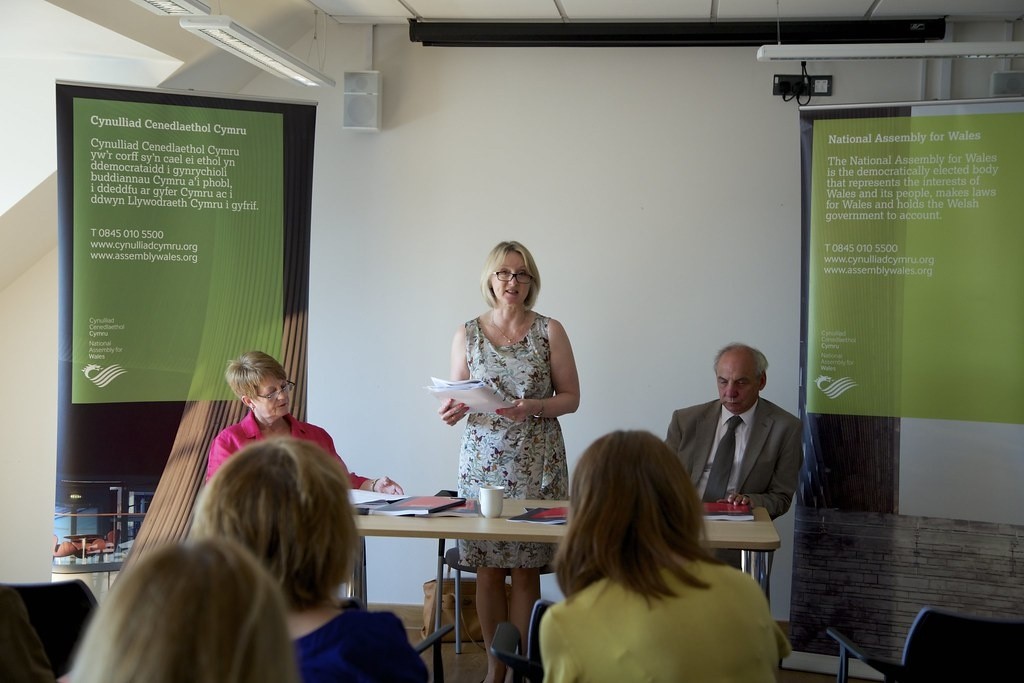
[
  {"left": 491, "top": 271, "right": 533, "bottom": 284},
  {"left": 256, "top": 381, "right": 295, "bottom": 400}
]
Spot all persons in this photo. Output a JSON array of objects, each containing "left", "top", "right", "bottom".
[
  {"left": 58, "top": 534, "right": 303, "bottom": 683},
  {"left": 438, "top": 239, "right": 580, "bottom": 683},
  {"left": 195, "top": 435, "right": 433, "bottom": 683},
  {"left": 664, "top": 343, "right": 805, "bottom": 567},
  {"left": 205, "top": 351, "right": 404, "bottom": 496},
  {"left": 0, "top": 584, "right": 57, "bottom": 683},
  {"left": 538, "top": 428, "right": 791, "bottom": 683}
]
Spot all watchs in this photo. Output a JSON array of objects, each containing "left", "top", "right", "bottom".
[{"left": 537, "top": 399, "right": 543, "bottom": 417}]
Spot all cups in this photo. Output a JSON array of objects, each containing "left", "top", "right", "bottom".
[{"left": 479, "top": 485, "right": 505, "bottom": 519}]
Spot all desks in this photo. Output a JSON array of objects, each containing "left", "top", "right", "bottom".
[
  {"left": 64, "top": 534, "right": 104, "bottom": 564},
  {"left": 350, "top": 496, "right": 780, "bottom": 683}
]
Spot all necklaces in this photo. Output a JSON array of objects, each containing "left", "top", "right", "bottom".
[{"left": 491, "top": 316, "right": 528, "bottom": 342}]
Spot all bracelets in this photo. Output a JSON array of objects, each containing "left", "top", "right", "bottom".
[{"left": 371, "top": 479, "right": 379, "bottom": 492}]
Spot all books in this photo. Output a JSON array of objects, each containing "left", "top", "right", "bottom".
[
  {"left": 352, "top": 491, "right": 480, "bottom": 518},
  {"left": 506, "top": 507, "right": 569, "bottom": 525},
  {"left": 701, "top": 502, "right": 754, "bottom": 520},
  {"left": 426, "top": 374, "right": 515, "bottom": 414}
]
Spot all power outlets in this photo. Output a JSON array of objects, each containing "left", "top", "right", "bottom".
[{"left": 772, "top": 74, "right": 833, "bottom": 97}]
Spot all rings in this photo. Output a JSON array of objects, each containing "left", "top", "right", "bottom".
[
  {"left": 512, "top": 416, "right": 514, "bottom": 419},
  {"left": 452, "top": 417, "right": 454, "bottom": 420}
]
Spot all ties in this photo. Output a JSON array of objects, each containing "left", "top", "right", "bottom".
[{"left": 702, "top": 415, "right": 743, "bottom": 503}]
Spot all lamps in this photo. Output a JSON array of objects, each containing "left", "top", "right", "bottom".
[
  {"left": 757, "top": 0, "right": 1024, "bottom": 61},
  {"left": 133, "top": 0, "right": 212, "bottom": 17},
  {"left": 180, "top": 0, "right": 336, "bottom": 87}
]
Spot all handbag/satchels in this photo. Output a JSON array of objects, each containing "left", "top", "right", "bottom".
[{"left": 421, "top": 578, "right": 511, "bottom": 651}]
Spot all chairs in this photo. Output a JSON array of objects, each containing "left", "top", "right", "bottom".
[
  {"left": 53, "top": 528, "right": 120, "bottom": 564},
  {"left": 434, "top": 535, "right": 556, "bottom": 654},
  {"left": 0, "top": 580, "right": 100, "bottom": 683},
  {"left": 825, "top": 604, "right": 1024, "bottom": 683}
]
